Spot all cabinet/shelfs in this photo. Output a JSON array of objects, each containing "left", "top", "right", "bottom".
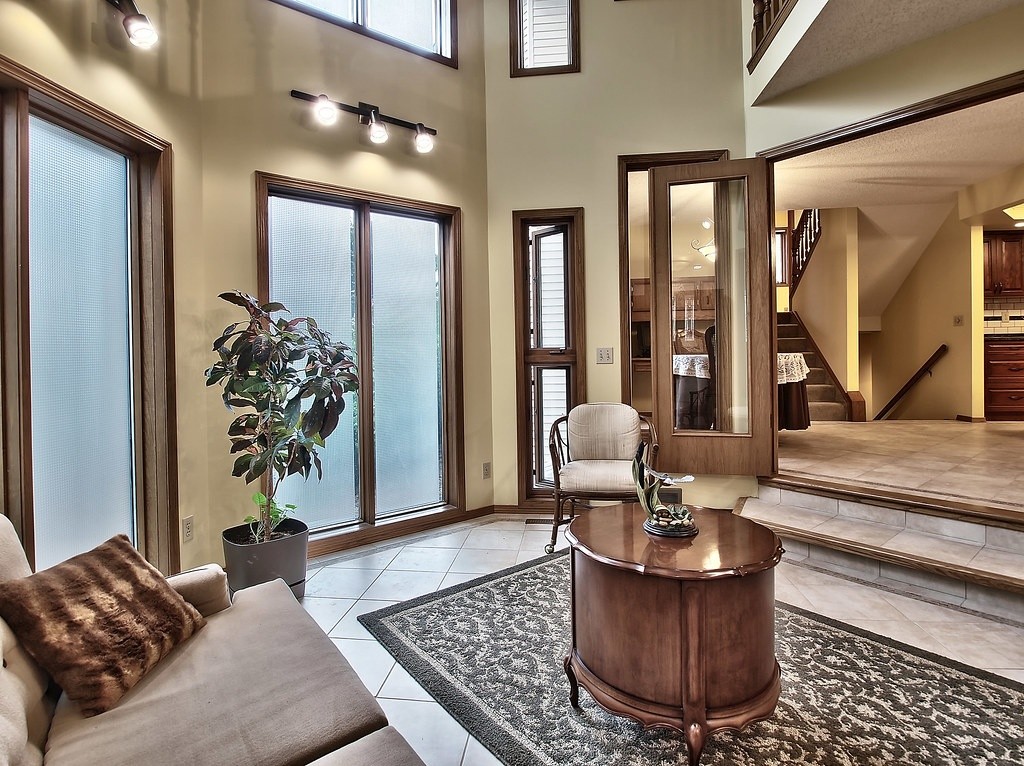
[
  {"left": 983, "top": 229, "right": 1024, "bottom": 298},
  {"left": 671, "top": 277, "right": 716, "bottom": 321},
  {"left": 984, "top": 333, "right": 1024, "bottom": 421},
  {"left": 631, "top": 277, "right": 652, "bottom": 321}
]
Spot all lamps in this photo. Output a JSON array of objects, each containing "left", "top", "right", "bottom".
[
  {"left": 313, "top": 93, "right": 339, "bottom": 126},
  {"left": 691, "top": 216, "right": 716, "bottom": 262},
  {"left": 368, "top": 109, "right": 388, "bottom": 143},
  {"left": 414, "top": 121, "right": 432, "bottom": 153},
  {"left": 109, "top": 1, "right": 158, "bottom": 47}
]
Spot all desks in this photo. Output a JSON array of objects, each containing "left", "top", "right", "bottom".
[
  {"left": 673, "top": 352, "right": 813, "bottom": 431},
  {"left": 563, "top": 502, "right": 786, "bottom": 766}
]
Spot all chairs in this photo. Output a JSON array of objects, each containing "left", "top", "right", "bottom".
[
  {"left": 544, "top": 401, "right": 659, "bottom": 553},
  {"left": 690, "top": 324, "right": 716, "bottom": 428}
]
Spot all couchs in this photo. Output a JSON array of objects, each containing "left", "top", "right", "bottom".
[{"left": 0, "top": 512, "right": 424, "bottom": 766}]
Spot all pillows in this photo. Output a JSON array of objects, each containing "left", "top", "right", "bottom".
[{"left": 0, "top": 535, "right": 207, "bottom": 718}]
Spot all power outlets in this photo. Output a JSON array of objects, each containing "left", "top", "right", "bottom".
[
  {"left": 482, "top": 462, "right": 491, "bottom": 479},
  {"left": 182, "top": 514, "right": 195, "bottom": 542},
  {"left": 1001, "top": 312, "right": 1010, "bottom": 323}
]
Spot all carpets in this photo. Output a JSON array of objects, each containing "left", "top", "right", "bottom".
[{"left": 357, "top": 545, "right": 1024, "bottom": 766}]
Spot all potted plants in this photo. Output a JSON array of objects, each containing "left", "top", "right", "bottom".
[{"left": 203, "top": 288, "right": 359, "bottom": 603}]
